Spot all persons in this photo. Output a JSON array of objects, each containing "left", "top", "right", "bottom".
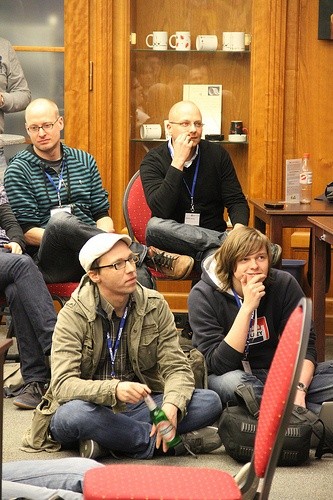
[
  {"left": 140, "top": 101, "right": 282, "bottom": 267},
  {"left": 23, "top": 233, "right": 222, "bottom": 459},
  {"left": 4, "top": 98, "right": 194, "bottom": 289},
  {"left": 187, "top": 227, "right": 333, "bottom": 406},
  {"left": 0, "top": 183, "right": 57, "bottom": 409},
  {"left": 0, "top": 36, "right": 32, "bottom": 186},
  {"left": 2, "top": 457, "right": 262, "bottom": 500}
]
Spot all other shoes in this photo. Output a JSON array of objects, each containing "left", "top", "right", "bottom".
[{"left": 268, "top": 243, "right": 283, "bottom": 267}]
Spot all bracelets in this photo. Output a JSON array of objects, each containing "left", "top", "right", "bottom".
[{"left": 1, "top": 97, "right": 4, "bottom": 107}]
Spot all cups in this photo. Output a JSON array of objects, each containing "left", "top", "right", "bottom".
[
  {"left": 140, "top": 124, "right": 162, "bottom": 140},
  {"left": 196, "top": 35, "right": 218, "bottom": 50},
  {"left": 146, "top": 31, "right": 168, "bottom": 51},
  {"left": 222, "top": 32, "right": 245, "bottom": 51},
  {"left": 169, "top": 31, "right": 191, "bottom": 51},
  {"left": 230, "top": 121, "right": 243, "bottom": 134}
]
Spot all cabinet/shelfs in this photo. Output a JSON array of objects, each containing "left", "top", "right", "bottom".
[{"left": 128, "top": 46, "right": 252, "bottom": 226}]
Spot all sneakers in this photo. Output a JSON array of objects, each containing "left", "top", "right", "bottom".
[
  {"left": 171, "top": 426, "right": 223, "bottom": 457},
  {"left": 78, "top": 439, "right": 103, "bottom": 459},
  {"left": 146, "top": 246, "right": 195, "bottom": 280},
  {"left": 13, "top": 381, "right": 49, "bottom": 409}
]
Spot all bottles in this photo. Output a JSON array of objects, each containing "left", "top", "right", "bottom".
[
  {"left": 298, "top": 153, "right": 313, "bottom": 204},
  {"left": 143, "top": 392, "right": 182, "bottom": 447}
]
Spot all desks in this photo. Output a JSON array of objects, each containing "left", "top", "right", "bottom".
[{"left": 248, "top": 198, "right": 333, "bottom": 363}]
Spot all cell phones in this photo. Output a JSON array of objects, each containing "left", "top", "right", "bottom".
[{"left": 264, "top": 203, "right": 284, "bottom": 209}]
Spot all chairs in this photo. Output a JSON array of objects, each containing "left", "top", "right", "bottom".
[
  {"left": 81, "top": 294, "right": 313, "bottom": 500},
  {"left": 3, "top": 280, "right": 79, "bottom": 368},
  {"left": 125, "top": 169, "right": 200, "bottom": 290}
]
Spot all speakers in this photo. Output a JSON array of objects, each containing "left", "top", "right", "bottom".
[{"left": 317, "top": 0, "right": 333, "bottom": 40}]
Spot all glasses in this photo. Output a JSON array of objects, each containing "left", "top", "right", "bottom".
[
  {"left": 27, "top": 115, "right": 61, "bottom": 133},
  {"left": 170, "top": 121, "right": 205, "bottom": 129},
  {"left": 95, "top": 255, "right": 139, "bottom": 270}
]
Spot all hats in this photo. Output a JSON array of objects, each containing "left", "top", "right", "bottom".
[{"left": 79, "top": 232, "right": 131, "bottom": 273}]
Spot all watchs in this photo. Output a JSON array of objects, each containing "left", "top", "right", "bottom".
[{"left": 297, "top": 382, "right": 308, "bottom": 393}]
